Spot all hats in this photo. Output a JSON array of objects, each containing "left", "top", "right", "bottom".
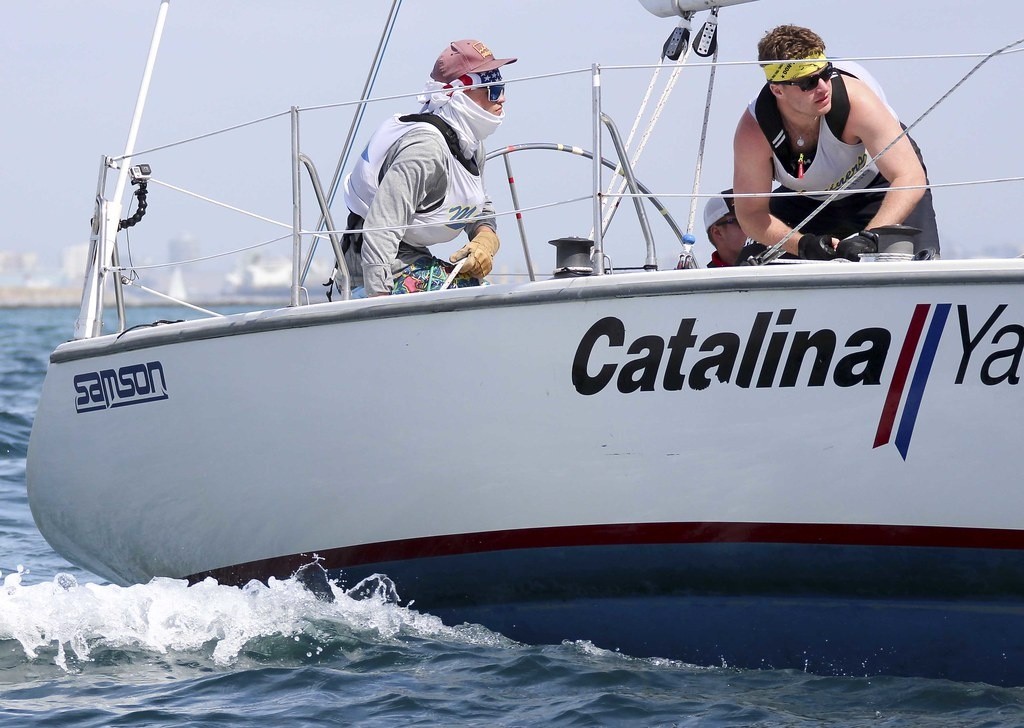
[
  {"left": 703, "top": 188, "right": 734, "bottom": 232},
  {"left": 430, "top": 40, "right": 518, "bottom": 83}
]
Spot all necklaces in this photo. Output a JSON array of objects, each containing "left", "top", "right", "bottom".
[{"left": 787, "top": 117, "right": 818, "bottom": 178}]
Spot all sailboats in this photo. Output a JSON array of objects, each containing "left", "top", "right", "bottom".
[{"left": 23, "top": 0, "right": 1024, "bottom": 687}]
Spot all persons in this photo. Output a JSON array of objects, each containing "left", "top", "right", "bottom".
[
  {"left": 733, "top": 24, "right": 941, "bottom": 262},
  {"left": 324, "top": 39, "right": 517, "bottom": 303},
  {"left": 703, "top": 189, "right": 747, "bottom": 268}
]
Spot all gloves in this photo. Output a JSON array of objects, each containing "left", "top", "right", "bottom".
[
  {"left": 448, "top": 232, "right": 500, "bottom": 277},
  {"left": 834, "top": 229, "right": 879, "bottom": 262}
]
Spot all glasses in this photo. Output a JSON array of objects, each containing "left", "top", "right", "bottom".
[
  {"left": 480, "top": 85, "right": 505, "bottom": 102},
  {"left": 768, "top": 61, "right": 834, "bottom": 92}
]
